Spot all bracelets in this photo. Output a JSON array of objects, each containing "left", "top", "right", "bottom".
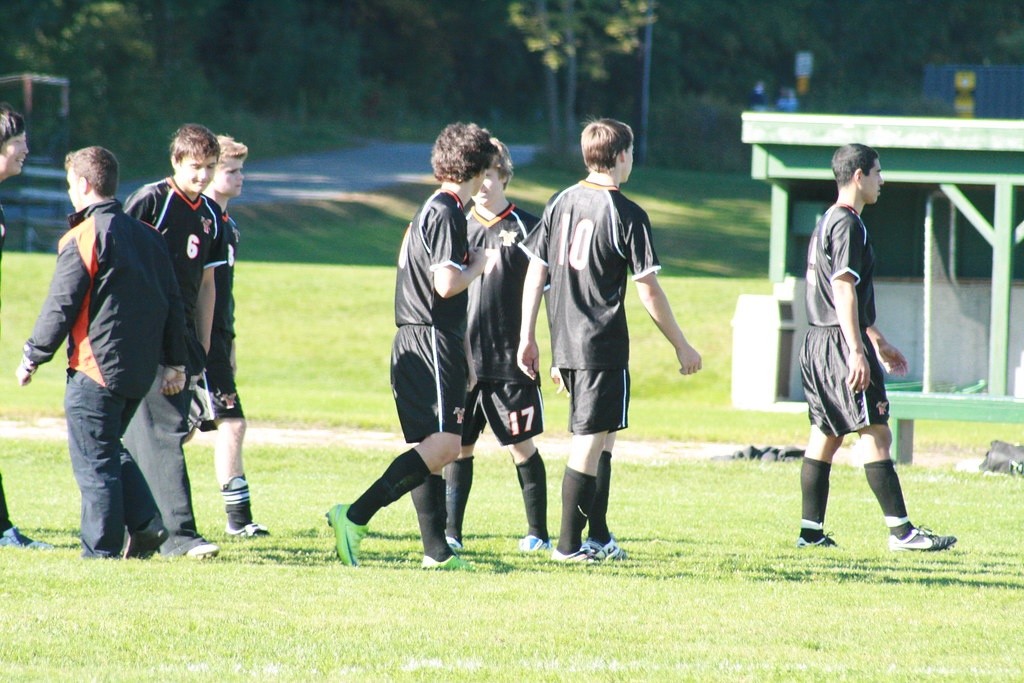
[{"left": 162, "top": 365, "right": 187, "bottom": 378}]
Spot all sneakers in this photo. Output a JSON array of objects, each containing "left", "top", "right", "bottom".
[
  {"left": 326, "top": 505, "right": 368, "bottom": 567},
  {"left": 423, "top": 554, "right": 465, "bottom": 570},
  {"left": 552, "top": 546, "right": 599, "bottom": 564},
  {"left": 184, "top": 544, "right": 221, "bottom": 559},
  {"left": 521, "top": 536, "right": 552, "bottom": 552},
  {"left": 796, "top": 532, "right": 840, "bottom": 547},
  {"left": 889, "top": 527, "right": 955, "bottom": 551},
  {"left": 224, "top": 523, "right": 269, "bottom": 538},
  {"left": 585, "top": 537, "right": 628, "bottom": 560},
  {"left": 445, "top": 535, "right": 463, "bottom": 550}
]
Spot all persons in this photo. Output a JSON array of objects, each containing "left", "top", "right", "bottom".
[
  {"left": 796, "top": 143, "right": 958, "bottom": 553},
  {"left": 0, "top": 100, "right": 55, "bottom": 551},
  {"left": 516, "top": 117, "right": 702, "bottom": 566},
  {"left": 322, "top": 120, "right": 565, "bottom": 566},
  {"left": 16, "top": 123, "right": 271, "bottom": 559}
]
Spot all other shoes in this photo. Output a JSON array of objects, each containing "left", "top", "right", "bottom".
[
  {"left": 79, "top": 515, "right": 169, "bottom": 559},
  {"left": 0, "top": 527, "right": 54, "bottom": 550}
]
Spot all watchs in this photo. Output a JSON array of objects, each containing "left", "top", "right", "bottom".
[{"left": 19, "top": 359, "right": 37, "bottom": 375}]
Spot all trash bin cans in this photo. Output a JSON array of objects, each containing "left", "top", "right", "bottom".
[{"left": 730, "top": 293, "right": 794, "bottom": 402}]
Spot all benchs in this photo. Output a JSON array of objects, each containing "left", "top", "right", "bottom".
[{"left": 886, "top": 389, "right": 1024, "bottom": 467}]
